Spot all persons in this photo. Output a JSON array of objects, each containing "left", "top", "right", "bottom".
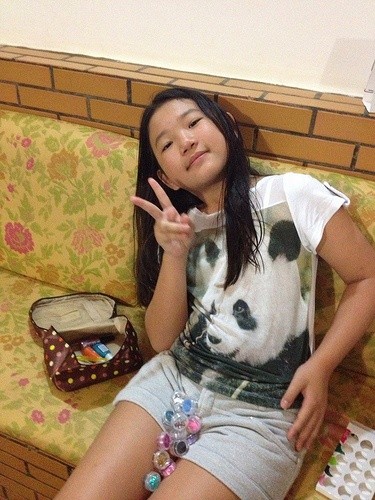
[{"left": 47, "top": 88, "right": 375, "bottom": 500}]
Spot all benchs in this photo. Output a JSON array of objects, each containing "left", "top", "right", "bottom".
[{"left": 0, "top": 111, "right": 375, "bottom": 500}]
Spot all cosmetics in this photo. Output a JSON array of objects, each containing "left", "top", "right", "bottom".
[{"left": 76, "top": 337, "right": 113, "bottom": 363}]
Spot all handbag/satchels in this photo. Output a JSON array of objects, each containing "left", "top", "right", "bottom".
[{"left": 28, "top": 291, "right": 144, "bottom": 394}]
